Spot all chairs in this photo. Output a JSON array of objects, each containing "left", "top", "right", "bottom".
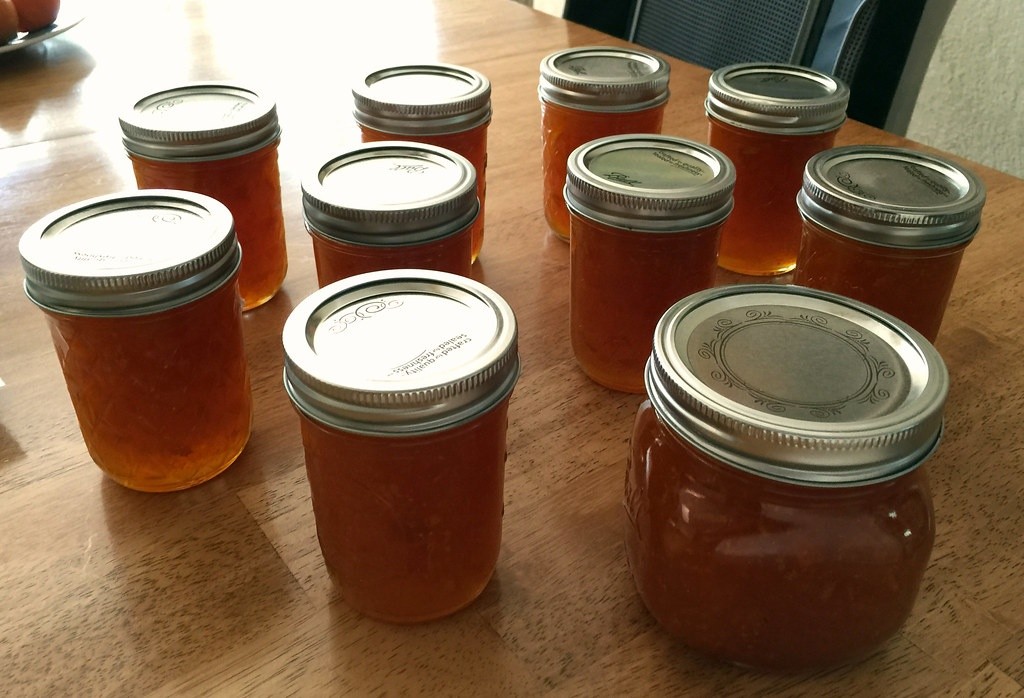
[{"left": 624, "top": 0, "right": 884, "bottom": 139}]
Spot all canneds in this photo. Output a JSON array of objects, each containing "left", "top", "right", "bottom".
[
  {"left": 350, "top": 63, "right": 492, "bottom": 263},
  {"left": 16, "top": 188, "right": 254, "bottom": 494},
  {"left": 704, "top": 61, "right": 850, "bottom": 276},
  {"left": 538, "top": 44, "right": 671, "bottom": 243},
  {"left": 563, "top": 133, "right": 736, "bottom": 394},
  {"left": 115, "top": 75, "right": 288, "bottom": 311},
  {"left": 620, "top": 282, "right": 951, "bottom": 667},
  {"left": 280, "top": 267, "right": 522, "bottom": 624},
  {"left": 300, "top": 141, "right": 480, "bottom": 289},
  {"left": 793, "top": 144, "right": 988, "bottom": 345}
]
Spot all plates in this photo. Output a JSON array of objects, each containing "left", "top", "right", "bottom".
[{"left": 0, "top": 15, "right": 90, "bottom": 53}]
